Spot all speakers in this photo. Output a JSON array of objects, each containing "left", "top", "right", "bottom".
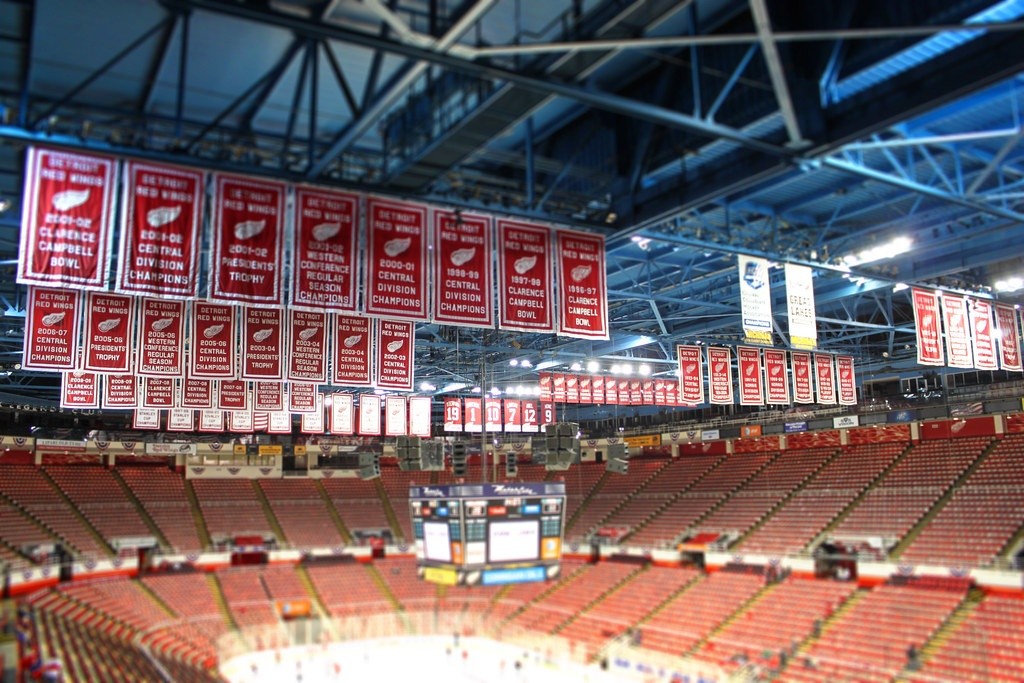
[{"left": 356, "top": 422, "right": 629, "bottom": 479}]
